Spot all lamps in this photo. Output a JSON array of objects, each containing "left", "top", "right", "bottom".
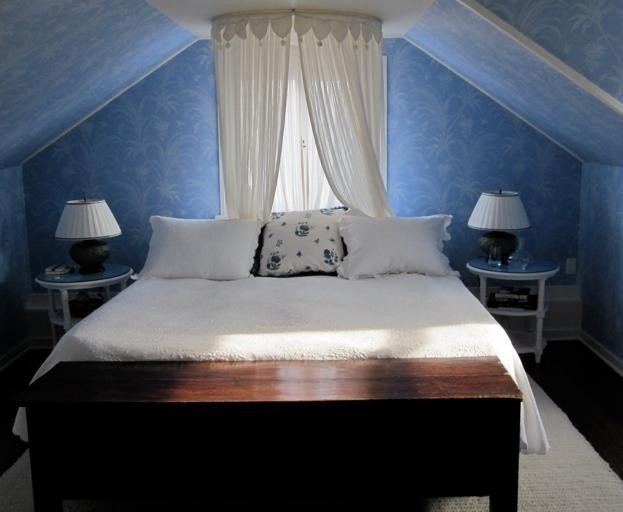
[
  {"left": 466, "top": 186, "right": 531, "bottom": 268},
  {"left": 54, "top": 196, "right": 123, "bottom": 276}
]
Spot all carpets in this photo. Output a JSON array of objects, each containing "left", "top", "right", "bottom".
[{"left": 0, "top": 371, "right": 622, "bottom": 512}]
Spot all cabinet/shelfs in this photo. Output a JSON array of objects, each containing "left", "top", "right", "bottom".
[
  {"left": 33, "top": 262, "right": 134, "bottom": 349},
  {"left": 470, "top": 255, "right": 561, "bottom": 364}
]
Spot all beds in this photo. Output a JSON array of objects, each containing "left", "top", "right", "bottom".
[{"left": 11, "top": 206, "right": 551, "bottom": 512}]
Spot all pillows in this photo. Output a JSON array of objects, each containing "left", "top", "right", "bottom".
[
  {"left": 335, "top": 213, "right": 454, "bottom": 278},
  {"left": 138, "top": 215, "right": 262, "bottom": 280},
  {"left": 257, "top": 219, "right": 347, "bottom": 278}
]
